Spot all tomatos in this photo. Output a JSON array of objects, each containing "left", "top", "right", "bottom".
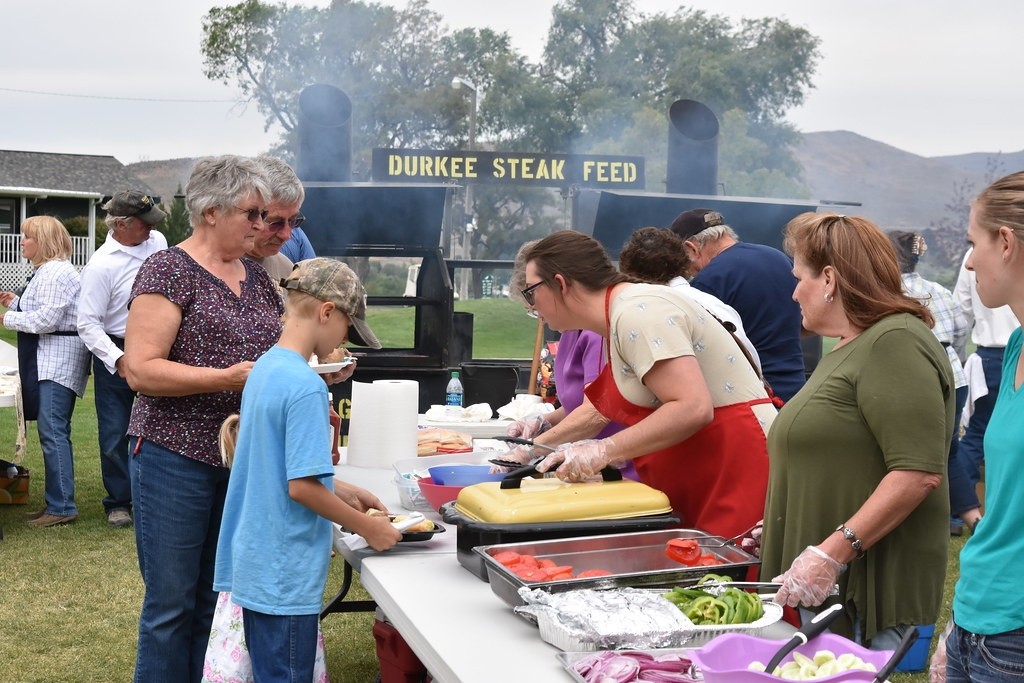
[
  {"left": 665, "top": 538, "right": 727, "bottom": 565},
  {"left": 491, "top": 551, "right": 613, "bottom": 584}
]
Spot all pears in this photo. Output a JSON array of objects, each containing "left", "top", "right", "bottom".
[{"left": 318, "top": 348, "right": 344, "bottom": 364}]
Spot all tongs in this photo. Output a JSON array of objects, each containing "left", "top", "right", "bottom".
[
  {"left": 678, "top": 524, "right": 764, "bottom": 548},
  {"left": 489, "top": 436, "right": 618, "bottom": 473},
  {"left": 682, "top": 581, "right": 839, "bottom": 601}
]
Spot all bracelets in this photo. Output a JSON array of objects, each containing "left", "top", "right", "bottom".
[
  {"left": 526, "top": 444, "right": 540, "bottom": 459},
  {"left": 836, "top": 524, "right": 865, "bottom": 559},
  {"left": 535, "top": 415, "right": 545, "bottom": 435}
]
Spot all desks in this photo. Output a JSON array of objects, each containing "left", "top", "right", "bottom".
[
  {"left": 0, "top": 338, "right": 21, "bottom": 542},
  {"left": 417, "top": 414, "right": 514, "bottom": 439}
]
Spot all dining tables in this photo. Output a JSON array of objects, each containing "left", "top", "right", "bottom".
[{"left": 320, "top": 445, "right": 893, "bottom": 683}]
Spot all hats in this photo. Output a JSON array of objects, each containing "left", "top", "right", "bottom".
[
  {"left": 106, "top": 189, "right": 167, "bottom": 226},
  {"left": 670, "top": 208, "right": 725, "bottom": 240},
  {"left": 279, "top": 257, "right": 382, "bottom": 350}
]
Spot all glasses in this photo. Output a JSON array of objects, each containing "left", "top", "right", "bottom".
[
  {"left": 521, "top": 275, "right": 555, "bottom": 306},
  {"left": 526, "top": 304, "right": 539, "bottom": 318},
  {"left": 233, "top": 205, "right": 269, "bottom": 222},
  {"left": 265, "top": 211, "right": 306, "bottom": 232}
]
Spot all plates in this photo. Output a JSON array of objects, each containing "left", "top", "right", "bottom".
[
  {"left": 340, "top": 517, "right": 446, "bottom": 542},
  {"left": 310, "top": 356, "right": 352, "bottom": 373}
]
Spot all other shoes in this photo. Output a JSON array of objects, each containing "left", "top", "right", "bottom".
[
  {"left": 108, "top": 510, "right": 133, "bottom": 527},
  {"left": 950, "top": 514, "right": 965, "bottom": 536},
  {"left": 970, "top": 518, "right": 980, "bottom": 535},
  {"left": 23, "top": 510, "right": 44, "bottom": 518},
  {"left": 27, "top": 513, "right": 77, "bottom": 527}
]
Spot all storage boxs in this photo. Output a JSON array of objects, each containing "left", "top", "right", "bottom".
[
  {"left": 373, "top": 606, "right": 428, "bottom": 683},
  {"left": 0, "top": 459, "right": 29, "bottom": 505},
  {"left": 440, "top": 463, "right": 681, "bottom": 581}
]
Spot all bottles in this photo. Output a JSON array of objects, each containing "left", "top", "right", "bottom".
[
  {"left": 327, "top": 393, "right": 341, "bottom": 465},
  {"left": 446, "top": 372, "right": 463, "bottom": 406}
]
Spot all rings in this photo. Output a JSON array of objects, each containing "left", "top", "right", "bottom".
[{"left": 571, "top": 472, "right": 577, "bottom": 477}]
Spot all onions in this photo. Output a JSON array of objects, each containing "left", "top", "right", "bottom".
[{"left": 572, "top": 650, "right": 700, "bottom": 683}]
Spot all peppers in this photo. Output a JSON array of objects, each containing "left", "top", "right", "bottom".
[{"left": 662, "top": 573, "right": 767, "bottom": 626}]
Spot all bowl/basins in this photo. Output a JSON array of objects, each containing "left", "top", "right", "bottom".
[
  {"left": 418, "top": 477, "right": 465, "bottom": 513},
  {"left": 394, "top": 474, "right": 434, "bottom": 511},
  {"left": 687, "top": 632, "right": 895, "bottom": 683},
  {"left": 428, "top": 466, "right": 510, "bottom": 486}
]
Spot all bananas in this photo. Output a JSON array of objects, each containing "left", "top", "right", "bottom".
[{"left": 746, "top": 650, "right": 877, "bottom": 680}]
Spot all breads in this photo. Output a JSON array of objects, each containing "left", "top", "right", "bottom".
[
  {"left": 394, "top": 515, "right": 435, "bottom": 531},
  {"left": 416, "top": 429, "right": 473, "bottom": 455}
]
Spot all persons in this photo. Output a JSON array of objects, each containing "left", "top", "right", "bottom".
[
  {"left": 201, "top": 413, "right": 326, "bottom": 683},
  {"left": 488, "top": 240, "right": 639, "bottom": 482},
  {"left": 620, "top": 227, "right": 762, "bottom": 371},
  {"left": 741, "top": 212, "right": 955, "bottom": 647},
  {"left": 279, "top": 226, "right": 316, "bottom": 264},
  {"left": 950, "top": 245, "right": 1021, "bottom": 537},
  {"left": 213, "top": 257, "right": 403, "bottom": 683},
  {"left": 77, "top": 188, "right": 169, "bottom": 528},
  {"left": 926, "top": 169, "right": 1024, "bottom": 683},
  {"left": 489, "top": 230, "right": 783, "bottom": 595},
  {"left": 886, "top": 228, "right": 984, "bottom": 535},
  {"left": 672, "top": 209, "right": 819, "bottom": 403},
  {"left": 244, "top": 155, "right": 357, "bottom": 384},
  {"left": 124, "top": 154, "right": 284, "bottom": 683},
  {"left": 0, "top": 215, "right": 93, "bottom": 526}
]
